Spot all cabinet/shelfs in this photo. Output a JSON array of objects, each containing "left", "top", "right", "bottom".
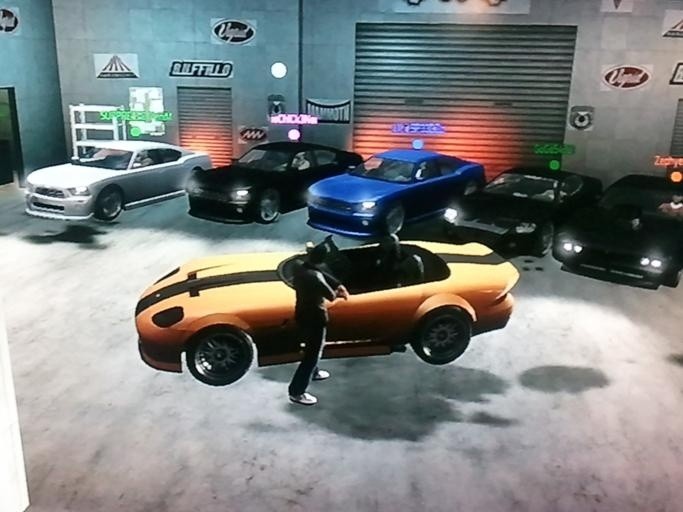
[{"left": 68, "top": 103, "right": 126, "bottom": 160}]
